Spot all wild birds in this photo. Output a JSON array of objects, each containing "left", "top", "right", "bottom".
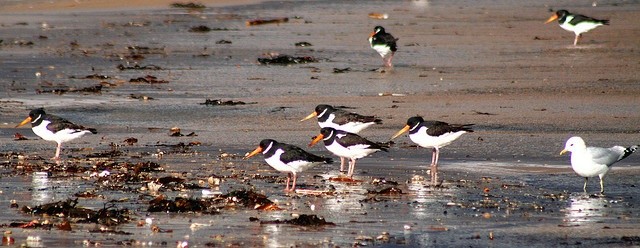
[
  {"left": 540, "top": 8, "right": 611, "bottom": 49},
  {"left": 298, "top": 104, "right": 383, "bottom": 134},
  {"left": 13, "top": 107, "right": 98, "bottom": 163},
  {"left": 242, "top": 138, "right": 335, "bottom": 198},
  {"left": 559, "top": 136, "right": 639, "bottom": 196},
  {"left": 366, "top": 24, "right": 402, "bottom": 72},
  {"left": 305, "top": 127, "right": 397, "bottom": 182},
  {"left": 390, "top": 116, "right": 476, "bottom": 169}
]
